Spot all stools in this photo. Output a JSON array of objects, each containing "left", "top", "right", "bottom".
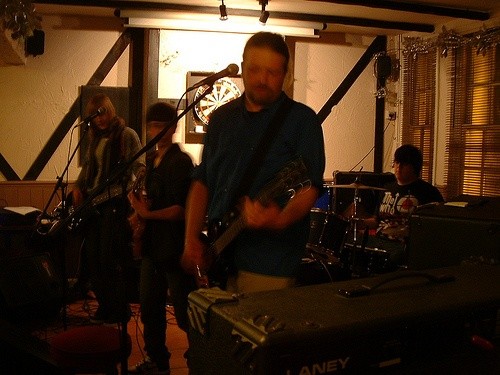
[{"left": 48, "top": 325, "right": 131, "bottom": 375}]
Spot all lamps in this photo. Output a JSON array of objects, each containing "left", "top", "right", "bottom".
[
  {"left": 259, "top": 0, "right": 271, "bottom": 26},
  {"left": 219, "top": 0, "right": 228, "bottom": 21}
]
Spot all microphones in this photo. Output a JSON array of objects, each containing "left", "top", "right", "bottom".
[
  {"left": 75, "top": 105, "right": 107, "bottom": 127},
  {"left": 192, "top": 64, "right": 239, "bottom": 89}
]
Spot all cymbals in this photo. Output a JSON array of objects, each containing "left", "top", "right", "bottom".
[{"left": 323, "top": 183, "right": 387, "bottom": 190}]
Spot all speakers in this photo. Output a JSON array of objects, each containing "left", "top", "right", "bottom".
[
  {"left": 377, "top": 56, "right": 391, "bottom": 80},
  {"left": 25, "top": 29, "right": 44, "bottom": 55},
  {"left": 187, "top": 256, "right": 500, "bottom": 375},
  {"left": 407, "top": 194, "right": 500, "bottom": 271}
]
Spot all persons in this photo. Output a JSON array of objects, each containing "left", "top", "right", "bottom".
[
  {"left": 348, "top": 144, "right": 445, "bottom": 273},
  {"left": 127, "top": 102, "right": 194, "bottom": 375},
  {"left": 180, "top": 33, "right": 327, "bottom": 292},
  {"left": 73, "top": 94, "right": 143, "bottom": 325}
]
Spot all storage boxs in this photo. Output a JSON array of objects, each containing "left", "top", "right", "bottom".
[
  {"left": 184, "top": 257, "right": 500, "bottom": 375},
  {"left": 410, "top": 196, "right": 500, "bottom": 268}
]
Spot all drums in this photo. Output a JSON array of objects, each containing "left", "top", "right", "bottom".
[{"left": 305, "top": 208, "right": 350, "bottom": 265}]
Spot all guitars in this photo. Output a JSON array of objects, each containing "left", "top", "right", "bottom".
[
  {"left": 128, "top": 173, "right": 151, "bottom": 260},
  {"left": 200, "top": 155, "right": 311, "bottom": 290},
  {"left": 65, "top": 163, "right": 146, "bottom": 232}
]
